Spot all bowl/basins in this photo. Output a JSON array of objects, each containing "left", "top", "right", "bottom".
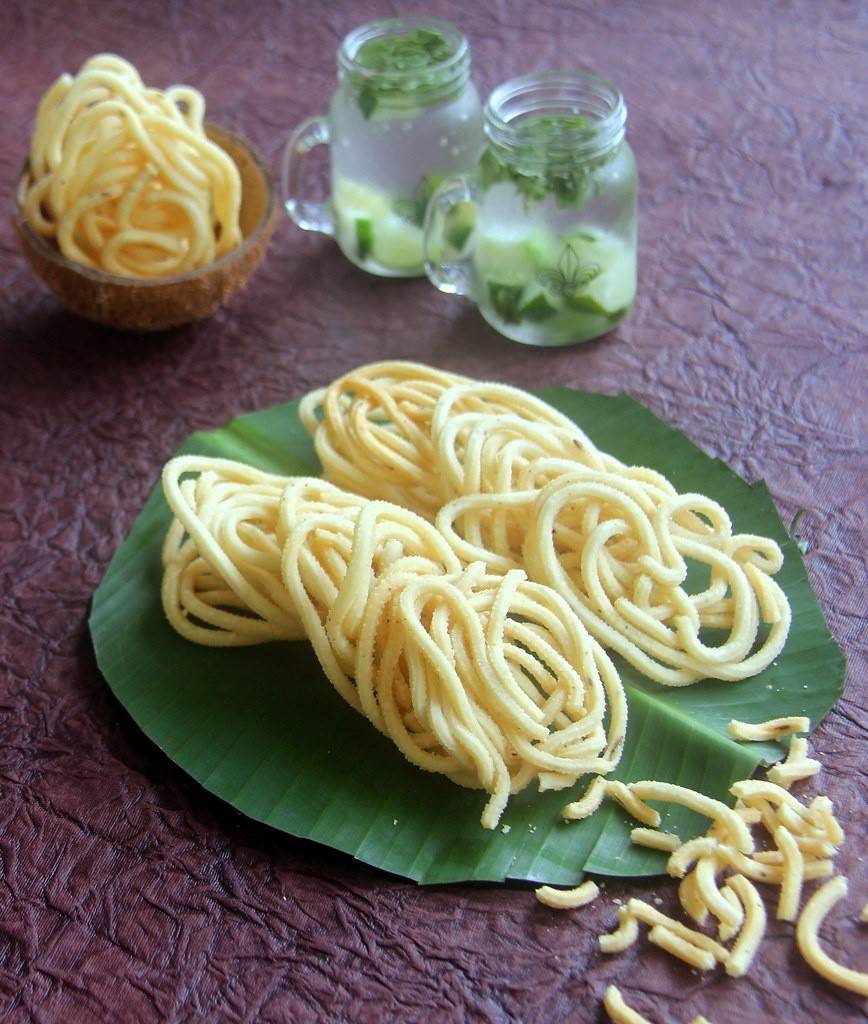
[{"left": 11, "top": 119, "right": 276, "bottom": 331}]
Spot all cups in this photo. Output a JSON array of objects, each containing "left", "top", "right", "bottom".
[
  {"left": 281, "top": 16, "right": 483, "bottom": 278},
  {"left": 420, "top": 70, "right": 641, "bottom": 350}
]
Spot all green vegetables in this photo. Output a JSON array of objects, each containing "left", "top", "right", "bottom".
[
  {"left": 345, "top": 27, "right": 462, "bottom": 121},
  {"left": 478, "top": 111, "right": 609, "bottom": 206}
]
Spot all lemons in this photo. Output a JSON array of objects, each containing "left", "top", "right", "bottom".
[{"left": 325, "top": 180, "right": 632, "bottom": 328}]
